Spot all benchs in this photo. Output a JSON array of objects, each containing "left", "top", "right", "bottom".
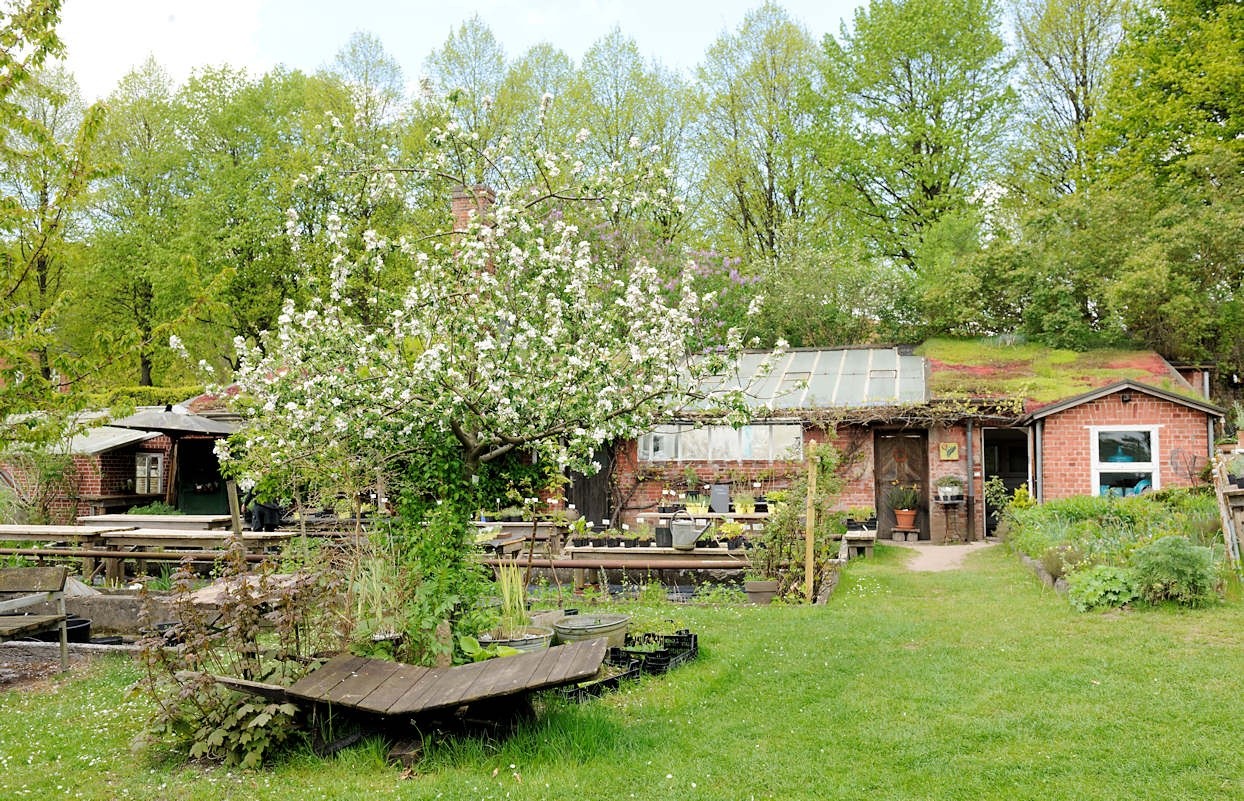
[
  {"left": 0, "top": 567, "right": 70, "bottom": 672},
  {"left": 845, "top": 521, "right": 879, "bottom": 560}
]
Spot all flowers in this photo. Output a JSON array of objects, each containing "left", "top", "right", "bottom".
[{"left": 889, "top": 479, "right": 919, "bottom": 509}]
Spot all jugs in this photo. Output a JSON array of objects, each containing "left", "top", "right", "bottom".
[{"left": 668, "top": 508, "right": 712, "bottom": 550}]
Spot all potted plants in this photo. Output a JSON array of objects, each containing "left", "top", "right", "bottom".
[
  {"left": 1217, "top": 400, "right": 1244, "bottom": 489},
  {"left": 935, "top": 477, "right": 964, "bottom": 502},
  {"left": 569, "top": 464, "right": 790, "bottom": 550},
  {"left": 742, "top": 541, "right": 780, "bottom": 603},
  {"left": 453, "top": 551, "right": 632, "bottom": 660},
  {"left": 846, "top": 505, "right": 876, "bottom": 530},
  {"left": 469, "top": 504, "right": 566, "bottom": 523}
]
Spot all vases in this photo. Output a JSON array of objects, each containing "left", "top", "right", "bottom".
[
  {"left": 893, "top": 509, "right": 918, "bottom": 530},
  {"left": 44, "top": 619, "right": 92, "bottom": 645},
  {"left": 89, "top": 636, "right": 124, "bottom": 647},
  {"left": 138, "top": 621, "right": 187, "bottom": 647}
]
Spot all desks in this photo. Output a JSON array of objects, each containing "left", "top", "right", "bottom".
[
  {"left": 567, "top": 545, "right": 773, "bottom": 597},
  {"left": 1221, "top": 483, "right": 1244, "bottom": 557},
  {"left": 0, "top": 514, "right": 569, "bottom": 611},
  {"left": 933, "top": 500, "right": 966, "bottom": 544}
]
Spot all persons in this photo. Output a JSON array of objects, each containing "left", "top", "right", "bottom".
[{"left": 238, "top": 489, "right": 282, "bottom": 532}]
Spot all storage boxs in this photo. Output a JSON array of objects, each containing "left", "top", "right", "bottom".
[
  {"left": 538, "top": 655, "right": 641, "bottom": 708},
  {"left": 609, "top": 632, "right": 698, "bottom": 679}
]
